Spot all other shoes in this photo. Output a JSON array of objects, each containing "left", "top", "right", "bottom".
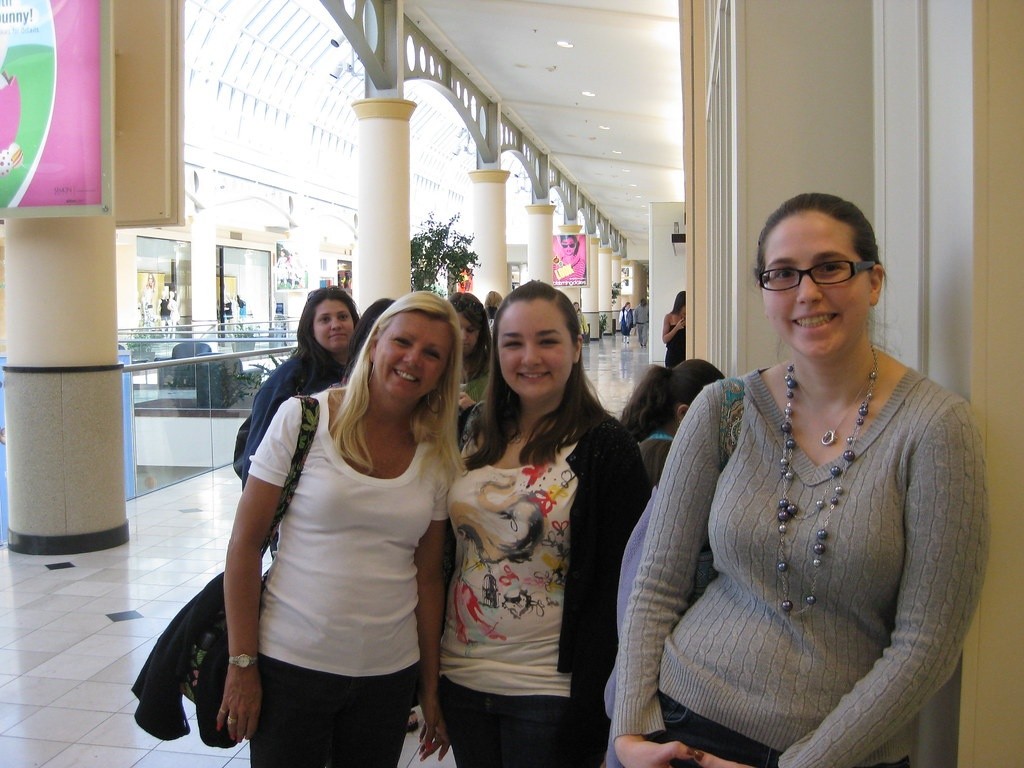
[{"left": 406, "top": 711, "right": 418, "bottom": 733}]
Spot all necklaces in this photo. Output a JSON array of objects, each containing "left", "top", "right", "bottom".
[{"left": 778, "top": 346, "right": 878, "bottom": 615}]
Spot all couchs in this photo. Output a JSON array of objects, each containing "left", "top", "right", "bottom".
[{"left": 154, "top": 342, "right": 212, "bottom": 390}]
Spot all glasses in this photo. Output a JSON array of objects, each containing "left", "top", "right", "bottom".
[
  {"left": 758, "top": 260, "right": 875, "bottom": 291},
  {"left": 451, "top": 300, "right": 483, "bottom": 311},
  {"left": 307, "top": 285, "right": 346, "bottom": 301}
]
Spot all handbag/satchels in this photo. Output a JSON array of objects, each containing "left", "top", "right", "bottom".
[
  {"left": 233, "top": 413, "right": 251, "bottom": 479},
  {"left": 180, "top": 578, "right": 264, "bottom": 747}
]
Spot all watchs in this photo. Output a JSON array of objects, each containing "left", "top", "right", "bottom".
[{"left": 229, "top": 655, "right": 259, "bottom": 668}]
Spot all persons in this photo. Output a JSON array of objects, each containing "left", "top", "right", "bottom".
[
  {"left": 483, "top": 291, "right": 503, "bottom": 338},
  {"left": 340, "top": 298, "right": 396, "bottom": 387},
  {"left": 633, "top": 298, "right": 649, "bottom": 348},
  {"left": 610, "top": 193, "right": 989, "bottom": 768},
  {"left": 433, "top": 280, "right": 726, "bottom": 768},
  {"left": 573, "top": 302, "right": 589, "bottom": 339},
  {"left": 274, "top": 249, "right": 303, "bottom": 289},
  {"left": 443, "top": 292, "right": 493, "bottom": 443},
  {"left": 158, "top": 290, "right": 180, "bottom": 340},
  {"left": 663, "top": 291, "right": 687, "bottom": 368},
  {"left": 619, "top": 302, "right": 633, "bottom": 343},
  {"left": 217, "top": 291, "right": 449, "bottom": 768},
  {"left": 143, "top": 273, "right": 156, "bottom": 318},
  {"left": 241, "top": 287, "right": 359, "bottom": 557},
  {"left": 621, "top": 359, "right": 724, "bottom": 487}
]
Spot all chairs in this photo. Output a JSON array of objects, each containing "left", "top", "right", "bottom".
[{"left": 118, "top": 344, "right": 148, "bottom": 385}]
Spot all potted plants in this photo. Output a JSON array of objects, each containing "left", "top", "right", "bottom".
[
  {"left": 612, "top": 281, "right": 622, "bottom": 334},
  {"left": 599, "top": 314, "right": 609, "bottom": 339},
  {"left": 127, "top": 334, "right": 155, "bottom": 376},
  {"left": 231, "top": 319, "right": 260, "bottom": 352}
]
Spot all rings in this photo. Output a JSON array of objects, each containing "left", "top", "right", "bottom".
[
  {"left": 227, "top": 718, "right": 237, "bottom": 724},
  {"left": 219, "top": 710, "right": 226, "bottom": 714}
]
